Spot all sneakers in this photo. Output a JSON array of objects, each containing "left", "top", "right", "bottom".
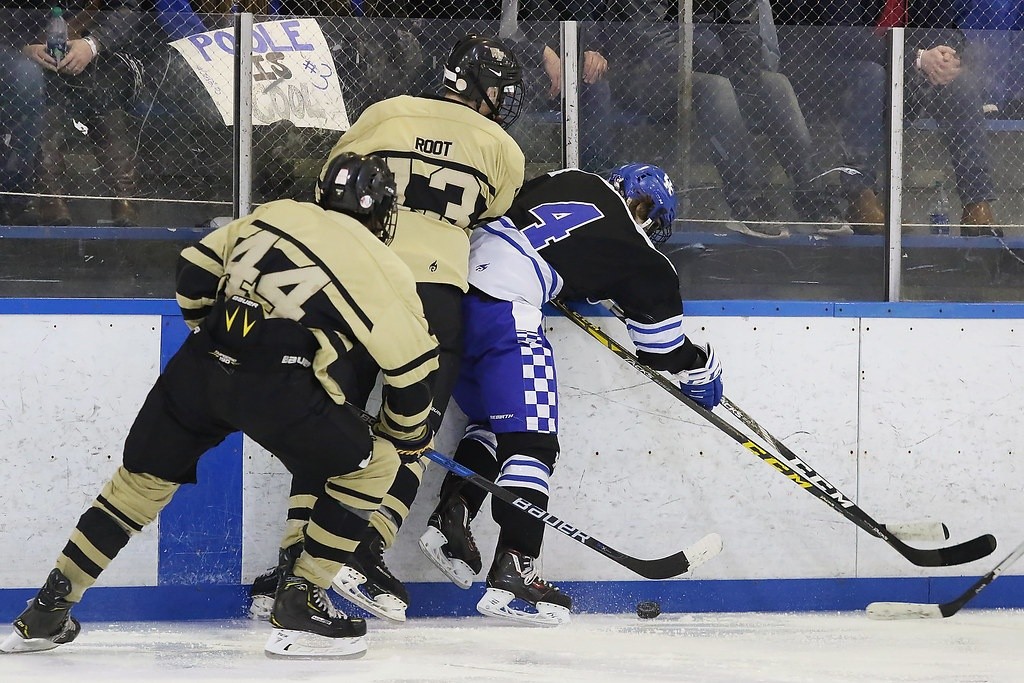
[
  {"left": 476, "top": 548, "right": 573, "bottom": 624},
  {"left": 418, "top": 493, "right": 483, "bottom": 590},
  {"left": 0, "top": 567, "right": 81, "bottom": 654},
  {"left": 249, "top": 564, "right": 278, "bottom": 621},
  {"left": 264, "top": 541, "right": 368, "bottom": 661},
  {"left": 331, "top": 526, "right": 411, "bottom": 624}
]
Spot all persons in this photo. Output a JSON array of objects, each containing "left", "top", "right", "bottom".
[
  {"left": 418, "top": 164, "right": 723, "bottom": 623},
  {"left": 0, "top": 151, "right": 438, "bottom": 660},
  {"left": 246, "top": 34, "right": 527, "bottom": 625},
  {"left": 0, "top": 0, "right": 1003, "bottom": 238}
]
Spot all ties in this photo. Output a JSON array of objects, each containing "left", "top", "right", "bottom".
[{"left": 874, "top": 1, "right": 905, "bottom": 38}]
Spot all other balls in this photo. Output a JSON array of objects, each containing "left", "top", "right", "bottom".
[{"left": 636, "top": 601, "right": 661, "bottom": 619}]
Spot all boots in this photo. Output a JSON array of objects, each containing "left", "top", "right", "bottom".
[
  {"left": 88, "top": 108, "right": 141, "bottom": 227},
  {"left": 34, "top": 129, "right": 74, "bottom": 225}
]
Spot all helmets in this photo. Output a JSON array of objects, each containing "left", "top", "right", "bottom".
[
  {"left": 442, "top": 32, "right": 526, "bottom": 130},
  {"left": 606, "top": 161, "right": 677, "bottom": 250},
  {"left": 316, "top": 151, "right": 399, "bottom": 246}
]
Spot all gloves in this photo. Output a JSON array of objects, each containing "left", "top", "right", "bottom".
[
  {"left": 370, "top": 418, "right": 436, "bottom": 465},
  {"left": 670, "top": 342, "right": 723, "bottom": 412}
]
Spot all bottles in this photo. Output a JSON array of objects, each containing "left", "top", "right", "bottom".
[
  {"left": 927, "top": 182, "right": 951, "bottom": 238},
  {"left": 45, "top": 8, "right": 68, "bottom": 60}
]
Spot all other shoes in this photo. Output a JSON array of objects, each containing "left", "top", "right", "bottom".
[
  {"left": 848, "top": 189, "right": 910, "bottom": 235},
  {"left": 959, "top": 199, "right": 1004, "bottom": 238},
  {"left": 816, "top": 216, "right": 854, "bottom": 235},
  {"left": 721, "top": 209, "right": 791, "bottom": 239}
]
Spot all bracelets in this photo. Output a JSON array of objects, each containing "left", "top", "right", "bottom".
[{"left": 916, "top": 48, "right": 923, "bottom": 69}]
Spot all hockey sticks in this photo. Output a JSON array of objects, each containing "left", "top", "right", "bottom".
[
  {"left": 547, "top": 297, "right": 1000, "bottom": 569},
  {"left": 345, "top": 400, "right": 691, "bottom": 581},
  {"left": 597, "top": 297, "right": 951, "bottom": 544},
  {"left": 862, "top": 542, "right": 1023, "bottom": 623}
]
